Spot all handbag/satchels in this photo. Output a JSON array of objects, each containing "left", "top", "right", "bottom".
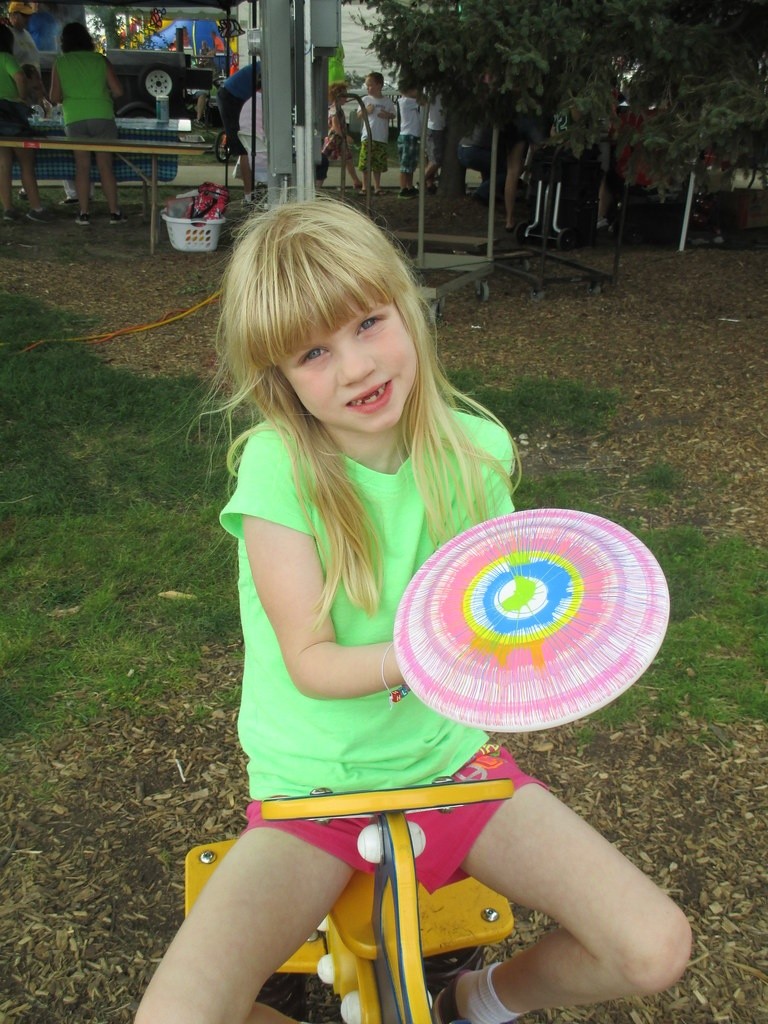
[{"left": 182, "top": 184, "right": 229, "bottom": 227}]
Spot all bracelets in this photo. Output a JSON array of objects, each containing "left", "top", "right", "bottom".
[{"left": 382, "top": 642, "right": 411, "bottom": 710}]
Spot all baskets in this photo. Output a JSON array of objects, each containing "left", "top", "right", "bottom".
[{"left": 160, "top": 205, "right": 225, "bottom": 253}]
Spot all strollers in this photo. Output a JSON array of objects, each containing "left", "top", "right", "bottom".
[
  {"left": 211, "top": 75, "right": 233, "bottom": 162},
  {"left": 514, "top": 143, "right": 649, "bottom": 252}
]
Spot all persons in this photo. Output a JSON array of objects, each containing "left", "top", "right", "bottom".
[
  {"left": 315, "top": 40, "right": 447, "bottom": 199},
  {"left": 452, "top": 51, "right": 733, "bottom": 251},
  {"left": 126, "top": 190, "right": 691, "bottom": 1024},
  {"left": 0, "top": 0, "right": 262, "bottom": 226}
]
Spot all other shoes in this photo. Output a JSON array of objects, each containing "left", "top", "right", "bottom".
[
  {"left": 353, "top": 182, "right": 363, "bottom": 190},
  {"left": 433, "top": 970, "right": 470, "bottom": 1024},
  {"left": 75, "top": 213, "right": 90, "bottom": 225},
  {"left": 2, "top": 210, "right": 19, "bottom": 221},
  {"left": 409, "top": 187, "right": 420, "bottom": 197},
  {"left": 373, "top": 189, "right": 386, "bottom": 195},
  {"left": 110, "top": 211, "right": 129, "bottom": 224},
  {"left": 194, "top": 121, "right": 205, "bottom": 129},
  {"left": 398, "top": 187, "right": 414, "bottom": 199},
  {"left": 26, "top": 208, "right": 57, "bottom": 224},
  {"left": 59, "top": 198, "right": 80, "bottom": 205},
  {"left": 417, "top": 181, "right": 436, "bottom": 192},
  {"left": 506, "top": 219, "right": 516, "bottom": 233},
  {"left": 358, "top": 189, "right": 367, "bottom": 196}
]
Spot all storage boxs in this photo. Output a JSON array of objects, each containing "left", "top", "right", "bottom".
[{"left": 161, "top": 207, "right": 226, "bottom": 252}]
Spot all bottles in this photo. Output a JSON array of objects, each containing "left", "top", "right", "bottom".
[{"left": 157, "top": 96, "right": 169, "bottom": 123}]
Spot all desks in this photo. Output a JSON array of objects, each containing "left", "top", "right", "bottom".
[
  {"left": 0, "top": 136, "right": 213, "bottom": 254},
  {"left": 13, "top": 117, "right": 192, "bottom": 210}
]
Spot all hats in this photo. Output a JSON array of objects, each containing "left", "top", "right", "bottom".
[{"left": 9, "top": 1, "right": 36, "bottom": 15}]
